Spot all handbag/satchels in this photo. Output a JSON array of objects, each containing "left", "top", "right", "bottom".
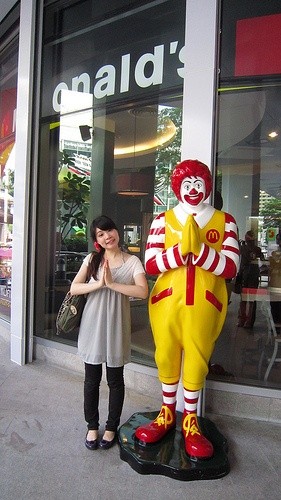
[{"left": 56, "top": 250, "right": 97, "bottom": 341}]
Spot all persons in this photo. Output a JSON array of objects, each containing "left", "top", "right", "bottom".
[
  {"left": 133, "top": 159, "right": 242, "bottom": 463},
  {"left": 236, "top": 231, "right": 281, "bottom": 333},
  {"left": 70, "top": 214, "right": 148, "bottom": 449}
]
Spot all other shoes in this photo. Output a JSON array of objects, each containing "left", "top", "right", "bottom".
[{"left": 231, "top": 273, "right": 242, "bottom": 294}]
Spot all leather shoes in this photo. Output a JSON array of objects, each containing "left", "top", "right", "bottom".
[
  {"left": 99, "top": 432, "right": 116, "bottom": 449},
  {"left": 85, "top": 430, "right": 96, "bottom": 450}
]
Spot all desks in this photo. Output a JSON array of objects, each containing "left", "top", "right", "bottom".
[{"left": 240, "top": 286, "right": 281, "bottom": 381}]
